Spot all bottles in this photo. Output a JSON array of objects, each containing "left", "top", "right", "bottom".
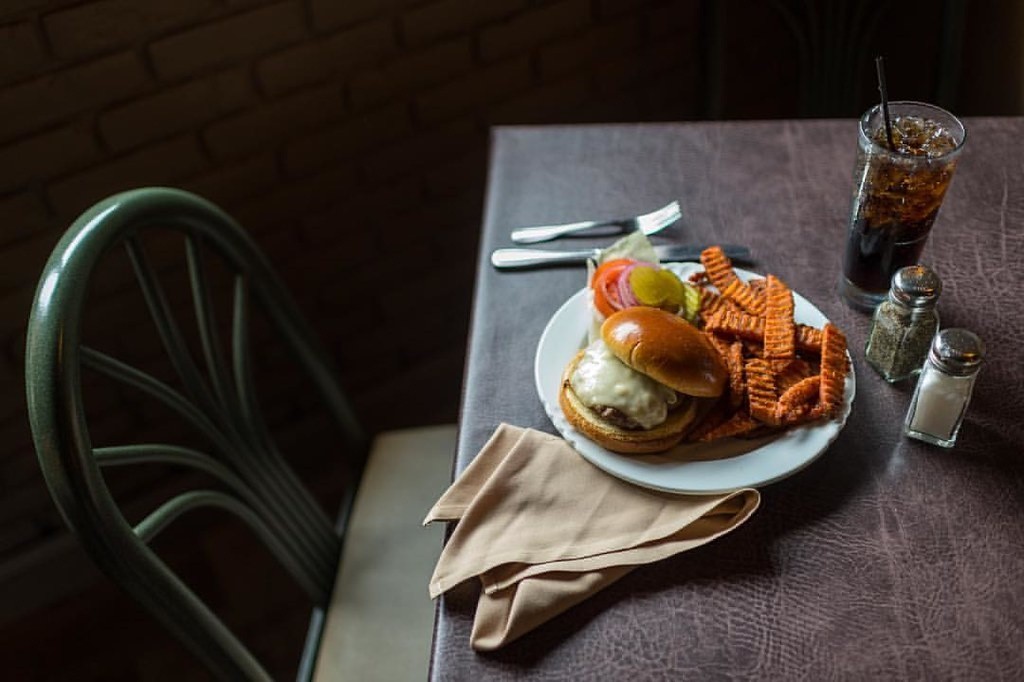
[{"left": 863, "top": 267, "right": 987, "bottom": 448}]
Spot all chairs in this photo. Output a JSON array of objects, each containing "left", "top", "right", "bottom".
[{"left": 24, "top": 187, "right": 457, "bottom": 682}]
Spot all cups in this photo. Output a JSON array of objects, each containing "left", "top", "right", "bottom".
[{"left": 840, "top": 101, "right": 967, "bottom": 312}]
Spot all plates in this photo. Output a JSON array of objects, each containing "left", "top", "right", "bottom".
[{"left": 535, "top": 262, "right": 859, "bottom": 496}]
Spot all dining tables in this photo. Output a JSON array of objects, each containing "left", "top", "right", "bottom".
[{"left": 429, "top": 114, "right": 1023, "bottom": 681}]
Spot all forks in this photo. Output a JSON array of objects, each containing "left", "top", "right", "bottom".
[{"left": 510, "top": 199, "right": 685, "bottom": 242}]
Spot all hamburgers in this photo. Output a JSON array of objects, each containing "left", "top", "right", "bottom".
[{"left": 557, "top": 302, "right": 731, "bottom": 454}]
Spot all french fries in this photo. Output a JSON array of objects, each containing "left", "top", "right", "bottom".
[{"left": 687, "top": 241, "right": 853, "bottom": 449}]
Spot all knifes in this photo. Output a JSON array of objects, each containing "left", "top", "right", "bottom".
[{"left": 490, "top": 243, "right": 752, "bottom": 267}]
